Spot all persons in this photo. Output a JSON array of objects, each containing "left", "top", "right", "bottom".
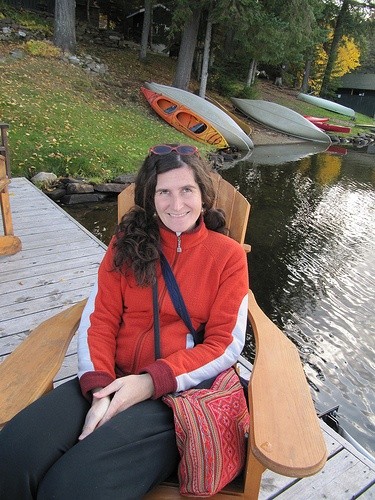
[{"left": 0, "top": 144, "right": 249, "bottom": 500}]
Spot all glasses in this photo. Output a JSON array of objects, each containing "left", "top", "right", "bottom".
[{"left": 148, "top": 145, "right": 200, "bottom": 158}]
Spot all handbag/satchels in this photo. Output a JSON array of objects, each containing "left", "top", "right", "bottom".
[{"left": 162, "top": 366, "right": 251, "bottom": 499}]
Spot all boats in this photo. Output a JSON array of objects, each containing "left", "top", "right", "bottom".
[
  {"left": 231, "top": 97, "right": 352, "bottom": 144},
  {"left": 140, "top": 82, "right": 256, "bottom": 154},
  {"left": 297, "top": 93, "right": 356, "bottom": 118}
]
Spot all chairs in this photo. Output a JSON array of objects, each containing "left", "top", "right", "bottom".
[{"left": 0, "top": 170, "right": 328, "bottom": 499}]
